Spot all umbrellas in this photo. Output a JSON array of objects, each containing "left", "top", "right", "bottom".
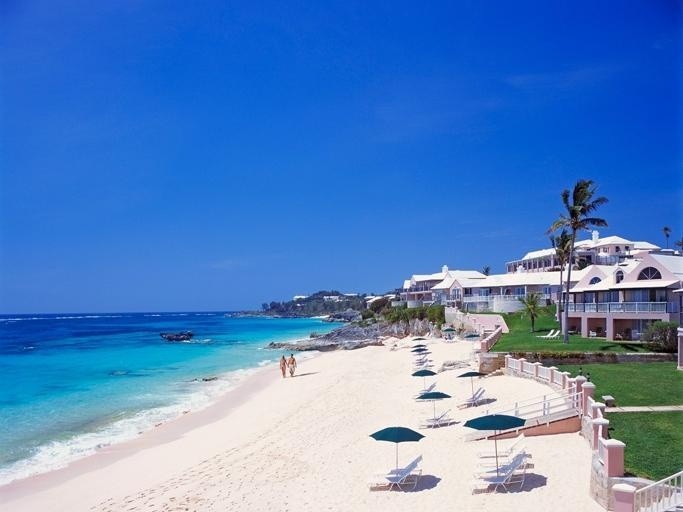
[{"left": 368, "top": 327, "right": 526, "bottom": 479}]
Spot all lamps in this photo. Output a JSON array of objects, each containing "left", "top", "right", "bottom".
[
  {"left": 586, "top": 372, "right": 591, "bottom": 382},
  {"left": 578, "top": 368, "right": 582, "bottom": 376}
]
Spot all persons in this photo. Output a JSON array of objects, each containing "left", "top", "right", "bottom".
[
  {"left": 287, "top": 354, "right": 296, "bottom": 377},
  {"left": 279, "top": 355, "right": 287, "bottom": 378}
]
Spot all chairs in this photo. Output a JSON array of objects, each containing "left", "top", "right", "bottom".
[
  {"left": 535, "top": 329, "right": 555, "bottom": 338},
  {"left": 465, "top": 387, "right": 485, "bottom": 407},
  {"left": 479, "top": 434, "right": 526, "bottom": 494},
  {"left": 418, "top": 382, "right": 436, "bottom": 395},
  {"left": 426, "top": 409, "right": 452, "bottom": 427},
  {"left": 385, "top": 455, "right": 422, "bottom": 493},
  {"left": 415, "top": 342, "right": 432, "bottom": 367},
  {"left": 441, "top": 335, "right": 458, "bottom": 343},
  {"left": 541, "top": 330, "right": 561, "bottom": 340}
]
